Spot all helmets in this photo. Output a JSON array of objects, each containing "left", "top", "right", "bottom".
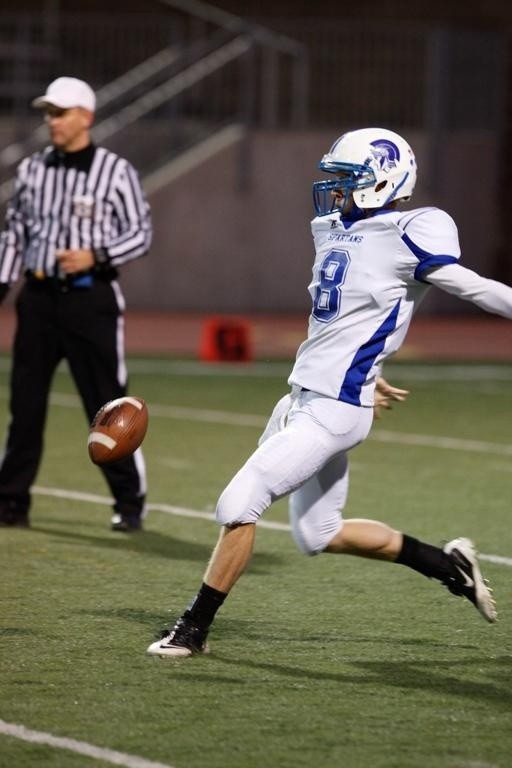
[{"left": 313, "top": 127, "right": 418, "bottom": 217}]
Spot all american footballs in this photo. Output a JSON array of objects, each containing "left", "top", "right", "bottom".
[{"left": 88, "top": 396, "right": 148, "bottom": 464}]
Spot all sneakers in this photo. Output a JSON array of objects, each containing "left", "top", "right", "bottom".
[
  {"left": 145, "top": 619, "right": 210, "bottom": 656},
  {"left": 446, "top": 537, "right": 497, "bottom": 624},
  {"left": 111, "top": 504, "right": 141, "bottom": 530}
]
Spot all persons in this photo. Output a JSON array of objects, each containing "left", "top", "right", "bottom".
[
  {"left": 147, "top": 125, "right": 512, "bottom": 658},
  {"left": 0, "top": 77, "right": 154, "bottom": 531}
]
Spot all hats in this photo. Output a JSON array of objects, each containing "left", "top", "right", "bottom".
[{"left": 34, "top": 75, "right": 97, "bottom": 114}]
